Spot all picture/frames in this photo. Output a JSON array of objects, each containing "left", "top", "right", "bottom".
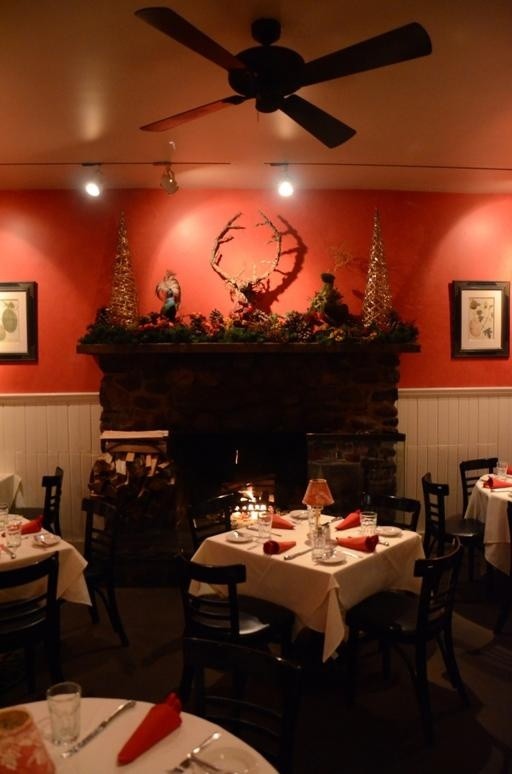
[
  {"left": 0, "top": 282, "right": 40, "bottom": 363},
  {"left": 451, "top": 280, "right": 510, "bottom": 359}
]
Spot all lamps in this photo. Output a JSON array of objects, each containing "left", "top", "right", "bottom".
[
  {"left": 158, "top": 163, "right": 178, "bottom": 195},
  {"left": 302, "top": 478, "right": 335, "bottom": 545},
  {"left": 84, "top": 163, "right": 106, "bottom": 197},
  {"left": 278, "top": 165, "right": 294, "bottom": 198},
  {"left": 0, "top": 709, "right": 56, "bottom": 773}
]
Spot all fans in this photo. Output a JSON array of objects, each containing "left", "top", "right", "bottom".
[{"left": 133, "top": 7, "right": 432, "bottom": 149}]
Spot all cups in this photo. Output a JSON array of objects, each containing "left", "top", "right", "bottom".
[
  {"left": 258, "top": 517, "right": 274, "bottom": 537},
  {"left": 497, "top": 461, "right": 508, "bottom": 479},
  {"left": 0, "top": 504, "right": 10, "bottom": 527},
  {"left": 5, "top": 523, "right": 22, "bottom": 547},
  {"left": 47, "top": 681, "right": 82, "bottom": 743},
  {"left": 360, "top": 512, "right": 378, "bottom": 535},
  {"left": 313, "top": 530, "right": 326, "bottom": 558},
  {"left": 191, "top": 747, "right": 257, "bottom": 774}
]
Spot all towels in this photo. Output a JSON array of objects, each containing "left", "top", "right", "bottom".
[
  {"left": 335, "top": 535, "right": 380, "bottom": 553},
  {"left": 505, "top": 465, "right": 512, "bottom": 474},
  {"left": 261, "top": 511, "right": 295, "bottom": 530},
  {"left": 117, "top": 693, "right": 183, "bottom": 763},
  {"left": 261, "top": 538, "right": 296, "bottom": 554},
  {"left": 482, "top": 476, "right": 512, "bottom": 488},
  {"left": 335, "top": 508, "right": 360, "bottom": 531},
  {"left": 1, "top": 514, "right": 43, "bottom": 537}
]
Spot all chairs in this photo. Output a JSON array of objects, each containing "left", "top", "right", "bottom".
[
  {"left": 0, "top": 551, "right": 62, "bottom": 704},
  {"left": 462, "top": 458, "right": 498, "bottom": 520},
  {"left": 344, "top": 534, "right": 472, "bottom": 748},
  {"left": 9, "top": 466, "right": 64, "bottom": 539},
  {"left": 166, "top": 548, "right": 296, "bottom": 713},
  {"left": 422, "top": 472, "right": 479, "bottom": 585},
  {"left": 178, "top": 636, "right": 305, "bottom": 773},
  {"left": 80, "top": 496, "right": 130, "bottom": 649},
  {"left": 376, "top": 495, "right": 421, "bottom": 531},
  {"left": 183, "top": 493, "right": 233, "bottom": 550}
]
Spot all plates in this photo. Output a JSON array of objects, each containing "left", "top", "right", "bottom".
[
  {"left": 377, "top": 526, "right": 401, "bottom": 536},
  {"left": 318, "top": 550, "right": 346, "bottom": 564},
  {"left": 227, "top": 532, "right": 253, "bottom": 543},
  {"left": 32, "top": 534, "right": 61, "bottom": 546},
  {"left": 290, "top": 509, "right": 310, "bottom": 519}
]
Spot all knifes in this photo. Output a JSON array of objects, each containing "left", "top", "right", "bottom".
[{"left": 60, "top": 701, "right": 136, "bottom": 759}]
